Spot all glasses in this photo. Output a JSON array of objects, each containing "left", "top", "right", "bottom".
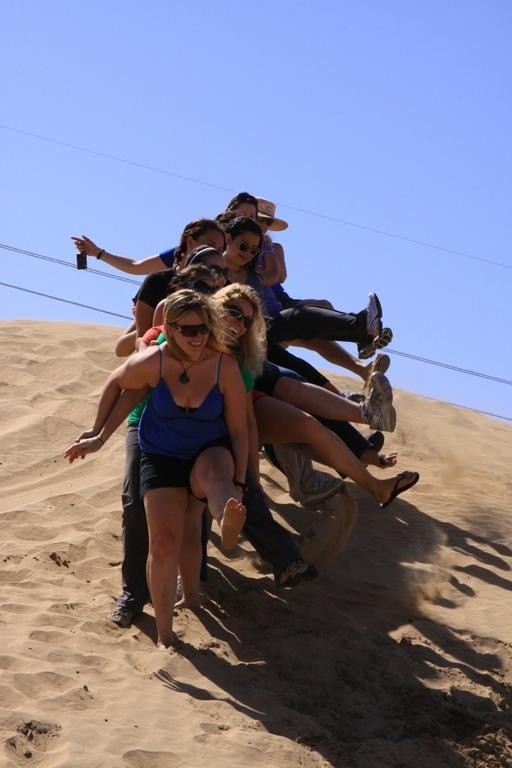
[
  {"left": 192, "top": 280, "right": 218, "bottom": 294},
  {"left": 208, "top": 265, "right": 232, "bottom": 281},
  {"left": 257, "top": 217, "right": 273, "bottom": 226},
  {"left": 235, "top": 239, "right": 260, "bottom": 256},
  {"left": 223, "top": 303, "right": 252, "bottom": 328},
  {"left": 170, "top": 322, "right": 208, "bottom": 336}
]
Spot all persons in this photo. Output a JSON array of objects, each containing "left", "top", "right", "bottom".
[{"left": 62, "top": 187, "right": 421, "bottom": 653}]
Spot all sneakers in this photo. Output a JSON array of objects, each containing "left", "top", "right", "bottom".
[
  {"left": 299, "top": 479, "right": 345, "bottom": 507},
  {"left": 366, "top": 292, "right": 382, "bottom": 337},
  {"left": 279, "top": 558, "right": 320, "bottom": 589},
  {"left": 359, "top": 328, "right": 392, "bottom": 359},
  {"left": 361, "top": 430, "right": 384, "bottom": 468},
  {"left": 360, "top": 371, "right": 396, "bottom": 432},
  {"left": 336, "top": 391, "right": 366, "bottom": 403},
  {"left": 176, "top": 576, "right": 183, "bottom": 596},
  {"left": 110, "top": 606, "right": 137, "bottom": 628}
]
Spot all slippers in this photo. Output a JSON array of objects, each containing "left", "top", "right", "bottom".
[
  {"left": 379, "top": 473, "right": 419, "bottom": 508},
  {"left": 365, "top": 354, "right": 390, "bottom": 387}
]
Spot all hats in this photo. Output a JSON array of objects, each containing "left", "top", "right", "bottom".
[{"left": 256, "top": 198, "right": 288, "bottom": 231}]
